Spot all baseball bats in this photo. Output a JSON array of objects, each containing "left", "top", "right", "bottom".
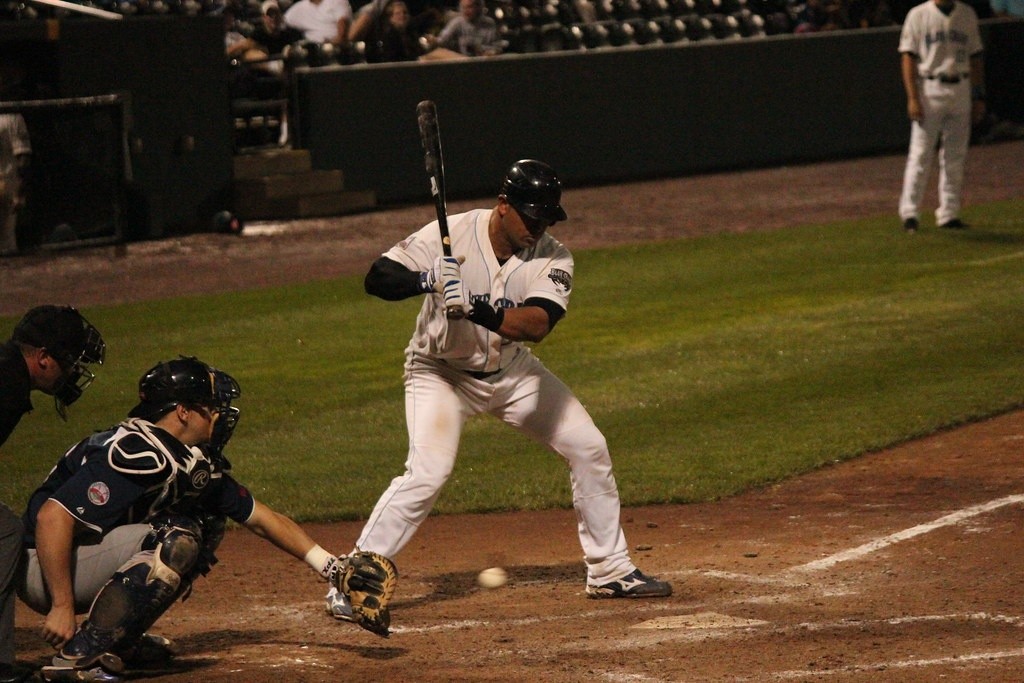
[{"left": 415, "top": 99, "right": 464, "bottom": 321}]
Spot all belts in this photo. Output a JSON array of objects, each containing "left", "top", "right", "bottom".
[{"left": 917, "top": 73, "right": 972, "bottom": 84}]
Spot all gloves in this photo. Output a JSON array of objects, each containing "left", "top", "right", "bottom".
[{"left": 421, "top": 255, "right": 474, "bottom": 318}]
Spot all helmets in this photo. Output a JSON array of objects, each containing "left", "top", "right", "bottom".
[
  {"left": 128, "top": 359, "right": 212, "bottom": 418},
  {"left": 501, "top": 161, "right": 568, "bottom": 222}
]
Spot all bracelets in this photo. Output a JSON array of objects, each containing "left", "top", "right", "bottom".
[{"left": 465, "top": 299, "right": 504, "bottom": 331}]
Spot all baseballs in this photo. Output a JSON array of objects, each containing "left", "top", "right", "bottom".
[{"left": 479, "top": 566, "right": 507, "bottom": 589}]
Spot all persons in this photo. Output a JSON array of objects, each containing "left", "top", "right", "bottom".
[
  {"left": 0, "top": 92, "right": 32, "bottom": 258},
  {"left": 0, "top": 304, "right": 107, "bottom": 683},
  {"left": 17, "top": 354, "right": 397, "bottom": 683},
  {"left": 209, "top": 0, "right": 501, "bottom": 146},
  {"left": 322, "top": 159, "right": 674, "bottom": 619},
  {"left": 989, "top": 0, "right": 1024, "bottom": 17},
  {"left": 898, "top": 0, "right": 983, "bottom": 233}
]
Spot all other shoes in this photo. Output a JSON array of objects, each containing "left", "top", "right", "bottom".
[
  {"left": 905, "top": 218, "right": 920, "bottom": 231},
  {"left": 0, "top": 661, "right": 30, "bottom": 683},
  {"left": 939, "top": 217, "right": 970, "bottom": 231}
]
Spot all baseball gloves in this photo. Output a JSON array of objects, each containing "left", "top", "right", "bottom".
[{"left": 333, "top": 552, "right": 398, "bottom": 637}]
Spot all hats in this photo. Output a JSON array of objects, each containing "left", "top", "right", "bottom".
[
  {"left": 260, "top": 0, "right": 281, "bottom": 16},
  {"left": 12, "top": 306, "right": 105, "bottom": 364}
]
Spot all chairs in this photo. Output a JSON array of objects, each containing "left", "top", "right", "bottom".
[{"left": 74, "top": 0, "right": 798, "bottom": 144}]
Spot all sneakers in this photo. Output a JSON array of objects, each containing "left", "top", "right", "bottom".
[
  {"left": 584, "top": 568, "right": 672, "bottom": 598},
  {"left": 327, "top": 604, "right": 353, "bottom": 622},
  {"left": 41, "top": 633, "right": 191, "bottom": 683}
]
